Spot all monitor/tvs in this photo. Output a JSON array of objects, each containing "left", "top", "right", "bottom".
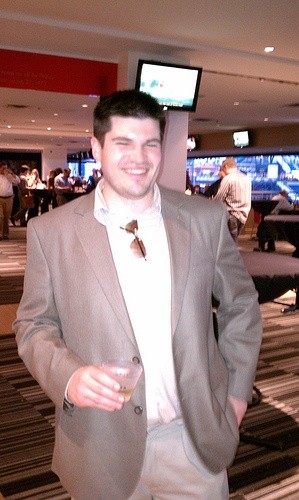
[
  {"left": 135, "top": 59, "right": 202, "bottom": 112},
  {"left": 187, "top": 136, "right": 198, "bottom": 150},
  {"left": 233, "top": 130, "right": 251, "bottom": 149}
]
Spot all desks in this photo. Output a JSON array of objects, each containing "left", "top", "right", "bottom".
[
  {"left": 213, "top": 251, "right": 299, "bottom": 407},
  {"left": 256, "top": 215, "right": 299, "bottom": 259},
  {"left": 18, "top": 181, "right": 86, "bottom": 220}
]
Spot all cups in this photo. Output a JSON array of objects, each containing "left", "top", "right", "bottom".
[{"left": 102, "top": 357, "right": 143, "bottom": 402}]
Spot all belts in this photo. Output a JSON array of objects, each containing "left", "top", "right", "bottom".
[{"left": 0, "top": 195, "right": 13, "bottom": 199}]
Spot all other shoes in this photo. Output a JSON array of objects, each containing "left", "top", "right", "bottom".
[
  {"left": 4, "top": 236, "right": 9, "bottom": 240},
  {"left": 20, "top": 221, "right": 27, "bottom": 227},
  {"left": 10, "top": 217, "right": 16, "bottom": 226},
  {"left": 0, "top": 237, "right": 3, "bottom": 241}
]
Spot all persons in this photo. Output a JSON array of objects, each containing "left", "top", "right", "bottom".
[
  {"left": 10, "top": 89, "right": 264, "bottom": 500},
  {"left": 0, "top": 156, "right": 299, "bottom": 242}
]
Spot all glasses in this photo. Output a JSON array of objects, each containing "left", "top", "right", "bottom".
[{"left": 119, "top": 220, "right": 147, "bottom": 261}]
